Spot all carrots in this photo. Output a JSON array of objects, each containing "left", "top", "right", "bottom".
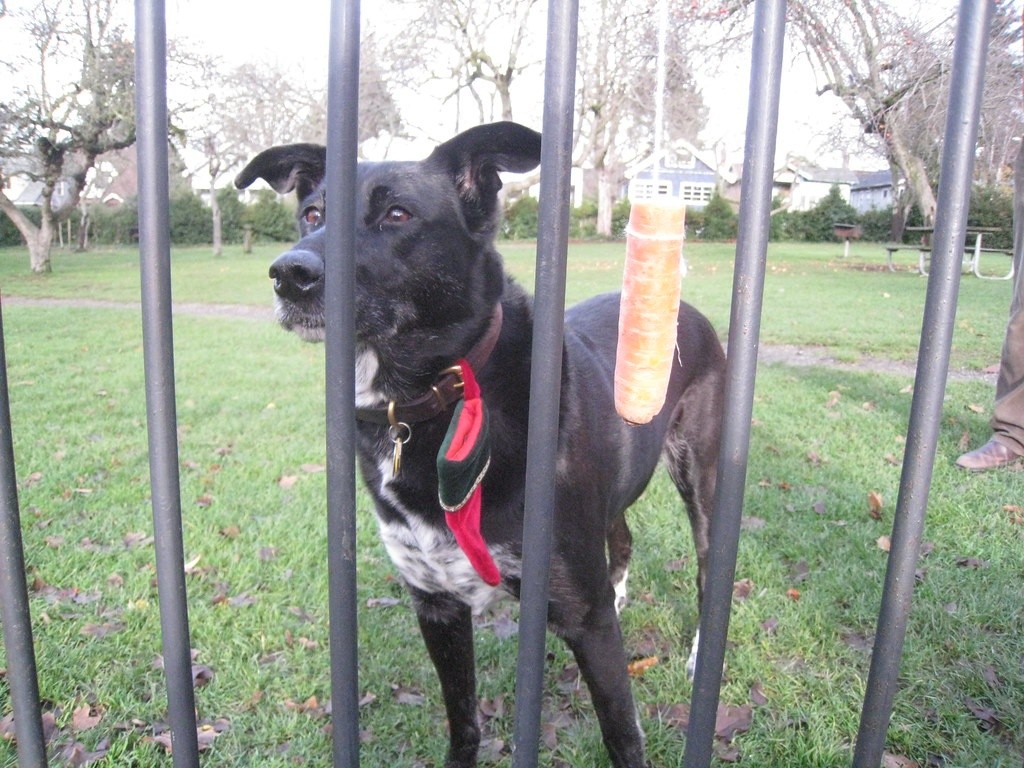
[{"left": 614, "top": 197, "right": 687, "bottom": 426}]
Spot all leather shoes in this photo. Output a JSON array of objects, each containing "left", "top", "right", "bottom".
[{"left": 955, "top": 440, "right": 1018, "bottom": 468}]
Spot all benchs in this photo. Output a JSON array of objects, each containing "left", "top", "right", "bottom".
[{"left": 884, "top": 245, "right": 1015, "bottom": 281}]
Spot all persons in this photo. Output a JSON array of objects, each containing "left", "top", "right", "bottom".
[{"left": 955, "top": 138, "right": 1024, "bottom": 469}]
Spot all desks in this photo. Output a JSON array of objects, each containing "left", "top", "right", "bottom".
[{"left": 906, "top": 225, "right": 1011, "bottom": 278}]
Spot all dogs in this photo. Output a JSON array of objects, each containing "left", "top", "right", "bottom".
[{"left": 233, "top": 121, "right": 727, "bottom": 767}]
